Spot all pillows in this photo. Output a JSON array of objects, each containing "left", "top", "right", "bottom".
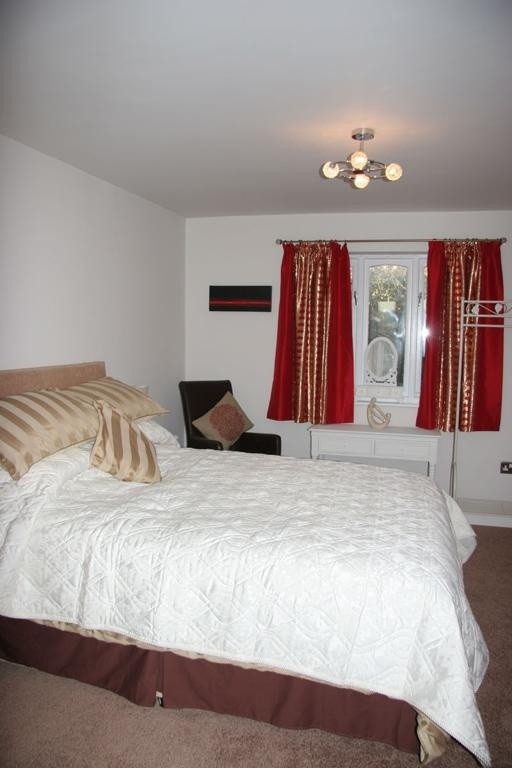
[
  {"left": 192, "top": 390, "right": 253, "bottom": 450},
  {"left": 65, "top": 376, "right": 172, "bottom": 422},
  {"left": 0, "top": 386, "right": 100, "bottom": 481},
  {"left": 91, "top": 398, "right": 163, "bottom": 484}
]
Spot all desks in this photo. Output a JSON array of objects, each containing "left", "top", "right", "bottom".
[{"left": 308, "top": 423, "right": 443, "bottom": 479}]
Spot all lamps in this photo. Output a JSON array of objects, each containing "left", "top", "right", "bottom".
[{"left": 322, "top": 127, "right": 403, "bottom": 189}]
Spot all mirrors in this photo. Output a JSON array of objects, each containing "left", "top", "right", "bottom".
[{"left": 363, "top": 337, "right": 399, "bottom": 386}]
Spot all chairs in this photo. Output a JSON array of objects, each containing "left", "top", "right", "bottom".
[{"left": 178, "top": 380, "right": 283, "bottom": 457}]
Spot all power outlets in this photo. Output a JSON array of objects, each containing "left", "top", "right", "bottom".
[{"left": 501, "top": 462, "right": 512, "bottom": 474}]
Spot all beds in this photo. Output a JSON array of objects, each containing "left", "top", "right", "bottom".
[{"left": 1, "top": 359, "right": 492, "bottom": 768}]
